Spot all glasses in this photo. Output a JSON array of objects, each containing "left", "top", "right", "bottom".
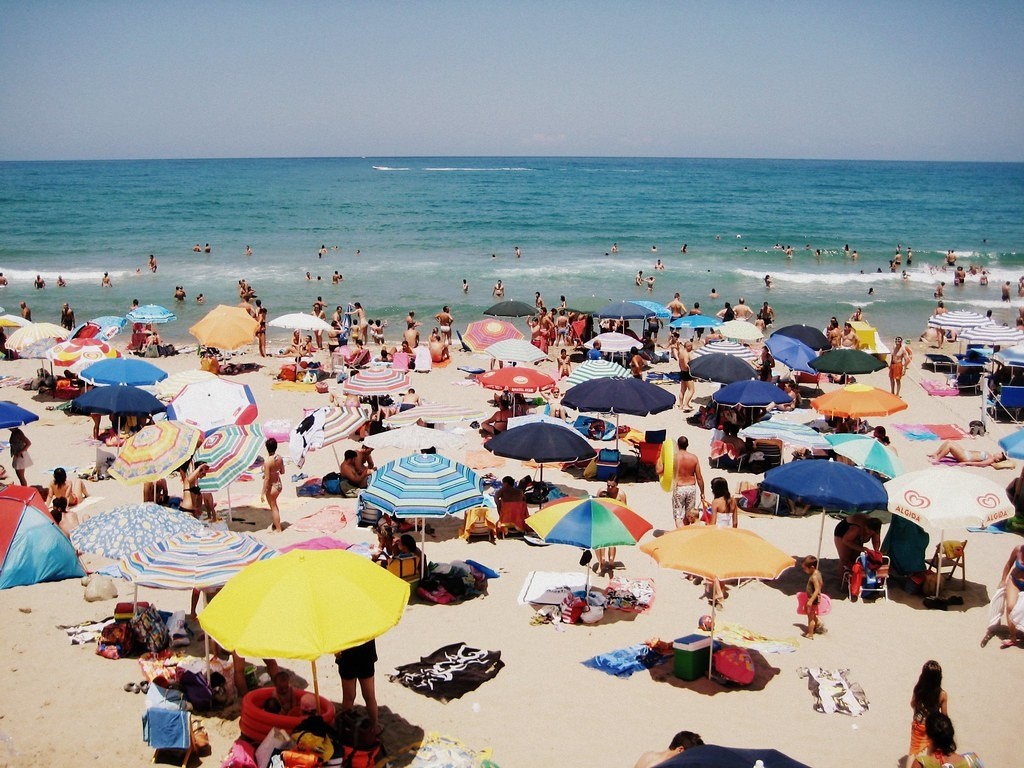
[{"left": 895, "top": 337, "right": 902, "bottom": 342}]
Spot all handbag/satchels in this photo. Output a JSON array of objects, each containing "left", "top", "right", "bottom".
[{"left": 255, "top": 727, "right": 291, "bottom": 768}]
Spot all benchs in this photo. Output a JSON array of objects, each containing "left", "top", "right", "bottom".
[
  {"left": 925, "top": 354, "right": 955, "bottom": 373},
  {"left": 794, "top": 372, "right": 821, "bottom": 390}
]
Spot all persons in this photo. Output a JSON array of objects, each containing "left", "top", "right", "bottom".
[
  {"left": 334, "top": 639, "right": 384, "bottom": 735},
  {"left": 906, "top": 662, "right": 984, "bottom": 768},
  {"left": 636, "top": 731, "right": 704, "bottom": 768},
  {"left": 2, "top": 235, "right": 1024, "bottom": 653}
]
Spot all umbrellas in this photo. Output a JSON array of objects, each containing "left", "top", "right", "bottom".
[
  {"left": 652, "top": 745, "right": 811, "bottom": 768},
  {"left": 0, "top": 300, "right": 1024, "bottom": 712}
]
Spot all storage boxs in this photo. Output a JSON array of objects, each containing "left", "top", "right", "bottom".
[
  {"left": 402, "top": 575, "right": 421, "bottom": 606},
  {"left": 596, "top": 461, "right": 621, "bottom": 481},
  {"left": 672, "top": 634, "right": 711, "bottom": 682}
]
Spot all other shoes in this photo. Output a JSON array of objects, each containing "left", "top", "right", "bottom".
[
  {"left": 924, "top": 598, "right": 947, "bottom": 611},
  {"left": 140, "top": 680, "right": 150, "bottom": 694},
  {"left": 291, "top": 473, "right": 308, "bottom": 482},
  {"left": 123, "top": 682, "right": 140, "bottom": 694},
  {"left": 942, "top": 595, "right": 963, "bottom": 606}
]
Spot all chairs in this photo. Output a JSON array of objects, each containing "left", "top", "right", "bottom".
[
  {"left": 458, "top": 507, "right": 496, "bottom": 545},
  {"left": 925, "top": 540, "right": 968, "bottom": 598},
  {"left": 714, "top": 440, "right": 747, "bottom": 472},
  {"left": 987, "top": 386, "right": 1024, "bottom": 421},
  {"left": 146, "top": 708, "right": 200, "bottom": 768},
  {"left": 496, "top": 489, "right": 529, "bottom": 539},
  {"left": 841, "top": 556, "right": 891, "bottom": 602},
  {"left": 953, "top": 344, "right": 984, "bottom": 360},
  {"left": 329, "top": 352, "right": 347, "bottom": 377}
]
